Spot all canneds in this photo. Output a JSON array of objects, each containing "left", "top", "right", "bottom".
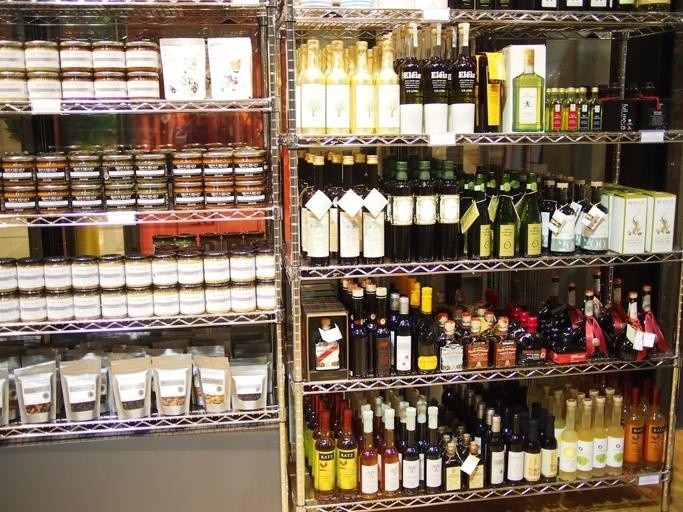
[
  {"left": 0, "top": 39, "right": 160, "bottom": 103},
  {"left": 1, "top": 141, "right": 267, "bottom": 209},
  {"left": 1, "top": 231, "right": 276, "bottom": 324}
]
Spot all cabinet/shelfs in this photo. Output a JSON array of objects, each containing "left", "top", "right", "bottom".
[
  {"left": 281, "top": 1, "right": 683, "bottom": 512},
  {"left": 0, "top": 2, "right": 281, "bottom": 441}
]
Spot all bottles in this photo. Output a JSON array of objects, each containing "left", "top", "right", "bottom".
[
  {"left": 295, "top": 148, "right": 388, "bottom": 264},
  {"left": 512, "top": 47, "right": 544, "bottom": 131},
  {"left": 457, "top": 163, "right": 542, "bottom": 260},
  {"left": 530, "top": 169, "right": 609, "bottom": 259},
  {"left": 304, "top": 381, "right": 668, "bottom": 500},
  {"left": 294, "top": 20, "right": 477, "bottom": 140},
  {"left": 314, "top": 318, "right": 340, "bottom": 371},
  {"left": 341, "top": 271, "right": 660, "bottom": 373},
  {"left": 385, "top": 155, "right": 459, "bottom": 260},
  {"left": 545, "top": 86, "right": 603, "bottom": 132},
  {"left": 598, "top": 82, "right": 663, "bottom": 131}
]
